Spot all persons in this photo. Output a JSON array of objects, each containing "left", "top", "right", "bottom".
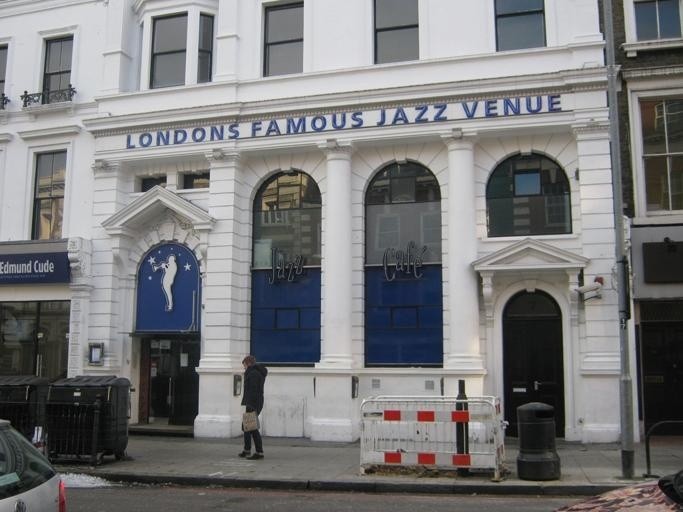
[
  {"left": 237, "top": 355, "right": 268, "bottom": 460},
  {"left": 158, "top": 254, "right": 177, "bottom": 312}
]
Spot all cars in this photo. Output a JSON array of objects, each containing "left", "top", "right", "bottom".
[{"left": 0, "top": 419, "right": 67, "bottom": 512}]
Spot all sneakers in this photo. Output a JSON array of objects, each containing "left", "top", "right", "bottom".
[
  {"left": 239, "top": 451, "right": 251, "bottom": 458},
  {"left": 247, "top": 453, "right": 264, "bottom": 460}
]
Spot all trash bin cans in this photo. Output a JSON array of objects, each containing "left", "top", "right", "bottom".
[
  {"left": 516, "top": 402, "right": 560, "bottom": 480},
  {"left": 0, "top": 375, "right": 132, "bottom": 465}
]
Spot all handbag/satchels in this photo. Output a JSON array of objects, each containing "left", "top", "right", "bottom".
[{"left": 243, "top": 411, "right": 260, "bottom": 432}]
